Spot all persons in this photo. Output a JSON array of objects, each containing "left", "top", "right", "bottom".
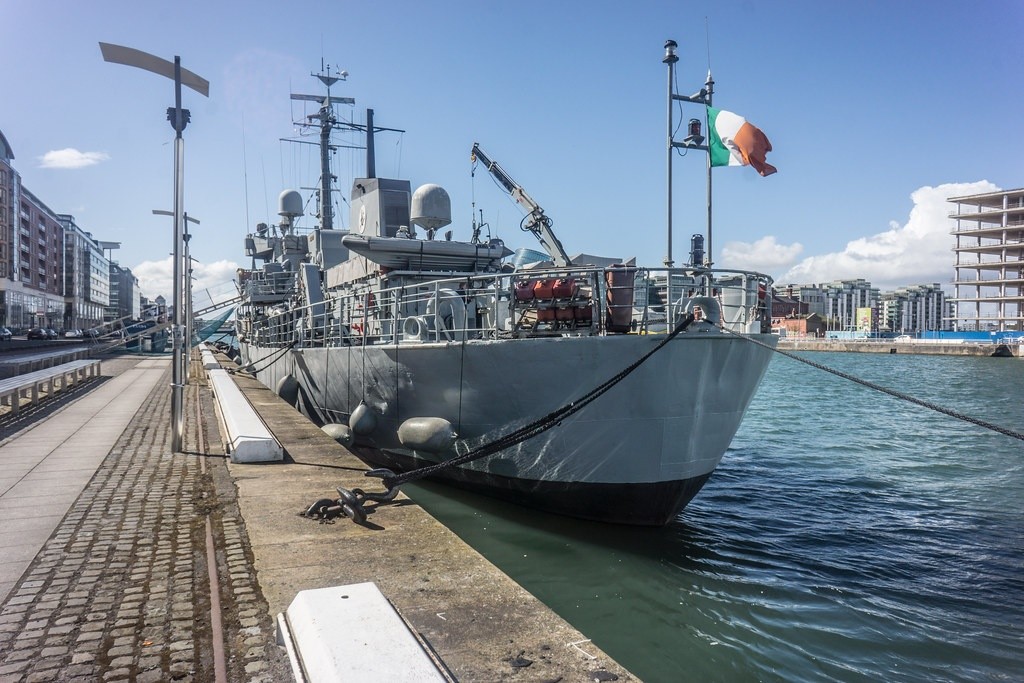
[
  {"left": 456, "top": 282, "right": 472, "bottom": 305},
  {"left": 476, "top": 287, "right": 487, "bottom": 334}
]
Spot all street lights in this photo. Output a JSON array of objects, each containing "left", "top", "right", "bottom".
[
  {"left": 151, "top": 207, "right": 201, "bottom": 385},
  {"left": 98, "top": 41, "right": 211, "bottom": 456}
]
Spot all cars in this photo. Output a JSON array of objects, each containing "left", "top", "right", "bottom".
[
  {"left": 894, "top": 335, "right": 913, "bottom": 342},
  {"left": 44, "top": 329, "right": 58, "bottom": 341},
  {"left": 27, "top": 328, "right": 48, "bottom": 341},
  {"left": 0, "top": 328, "right": 12, "bottom": 342},
  {"left": 1017, "top": 336, "right": 1024, "bottom": 343},
  {"left": 83, "top": 329, "right": 99, "bottom": 338},
  {"left": 65, "top": 330, "right": 83, "bottom": 339},
  {"left": 854, "top": 335, "right": 870, "bottom": 343}
]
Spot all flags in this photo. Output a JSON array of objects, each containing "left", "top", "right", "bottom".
[{"left": 707, "top": 107, "right": 778, "bottom": 177}]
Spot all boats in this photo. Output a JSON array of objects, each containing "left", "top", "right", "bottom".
[{"left": 231, "top": 40, "right": 777, "bottom": 532}]
